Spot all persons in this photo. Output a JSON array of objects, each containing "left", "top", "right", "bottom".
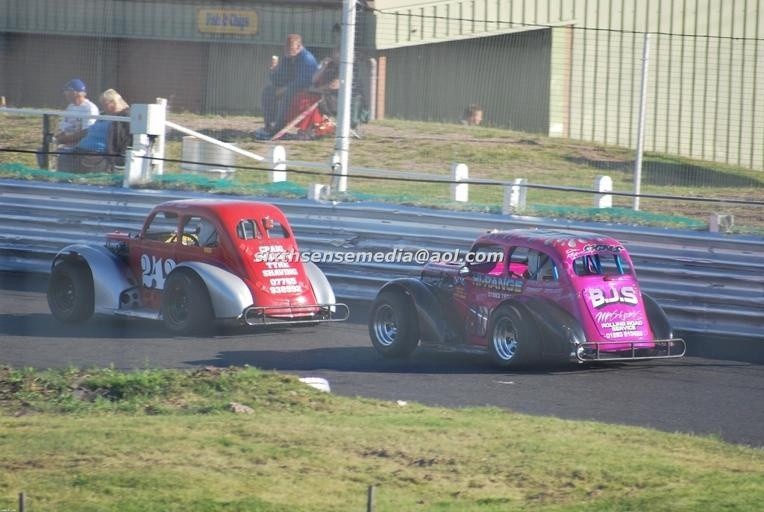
[
  {"left": 58, "top": 89, "right": 134, "bottom": 184},
  {"left": 194, "top": 218, "right": 218, "bottom": 247},
  {"left": 458, "top": 102, "right": 483, "bottom": 127},
  {"left": 289, "top": 43, "right": 366, "bottom": 139},
  {"left": 33, "top": 77, "right": 100, "bottom": 184},
  {"left": 525, "top": 246, "right": 554, "bottom": 281},
  {"left": 253, "top": 30, "right": 320, "bottom": 142}
]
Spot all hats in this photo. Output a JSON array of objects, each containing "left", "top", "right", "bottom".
[{"left": 59, "top": 79, "right": 88, "bottom": 92}]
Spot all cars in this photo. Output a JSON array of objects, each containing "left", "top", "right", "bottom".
[
  {"left": 46, "top": 198, "right": 350, "bottom": 334},
  {"left": 365, "top": 231, "right": 688, "bottom": 369}
]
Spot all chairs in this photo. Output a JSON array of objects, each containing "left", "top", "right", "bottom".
[
  {"left": 527, "top": 249, "right": 554, "bottom": 279},
  {"left": 198, "top": 217, "right": 217, "bottom": 248}
]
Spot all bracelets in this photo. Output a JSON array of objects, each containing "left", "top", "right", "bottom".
[{"left": 322, "top": 59, "right": 329, "bottom": 65}]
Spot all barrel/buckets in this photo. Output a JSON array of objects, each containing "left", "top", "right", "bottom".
[{"left": 180, "top": 136, "right": 235, "bottom": 180}]
